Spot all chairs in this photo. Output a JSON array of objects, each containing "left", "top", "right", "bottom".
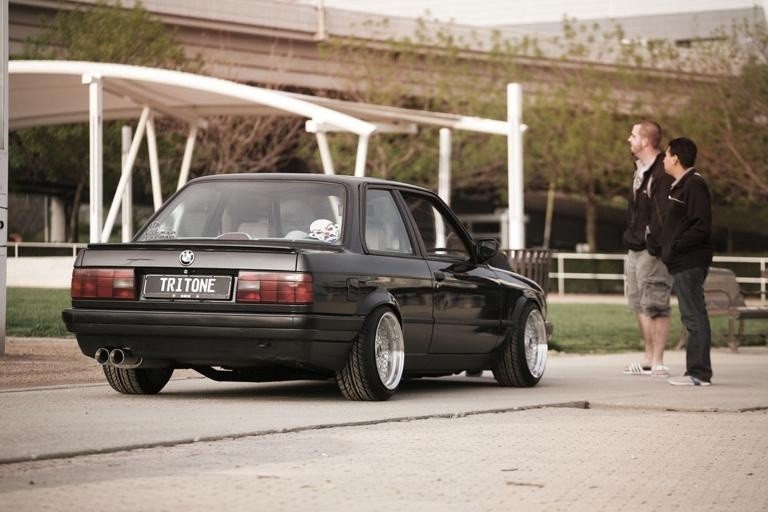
[{"left": 667, "top": 374, "right": 711, "bottom": 385}]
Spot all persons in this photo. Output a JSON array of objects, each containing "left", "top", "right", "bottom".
[
  {"left": 660, "top": 137, "right": 714, "bottom": 387},
  {"left": 619, "top": 119, "right": 676, "bottom": 380}
]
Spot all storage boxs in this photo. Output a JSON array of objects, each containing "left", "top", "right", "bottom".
[{"left": 671, "top": 266, "right": 768, "bottom": 354}]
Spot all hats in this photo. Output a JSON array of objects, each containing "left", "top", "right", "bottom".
[{"left": 57, "top": 170, "right": 554, "bottom": 403}]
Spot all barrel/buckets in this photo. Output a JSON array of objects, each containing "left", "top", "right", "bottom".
[
  {"left": 651, "top": 364, "right": 671, "bottom": 379},
  {"left": 622, "top": 361, "right": 653, "bottom": 374}
]
